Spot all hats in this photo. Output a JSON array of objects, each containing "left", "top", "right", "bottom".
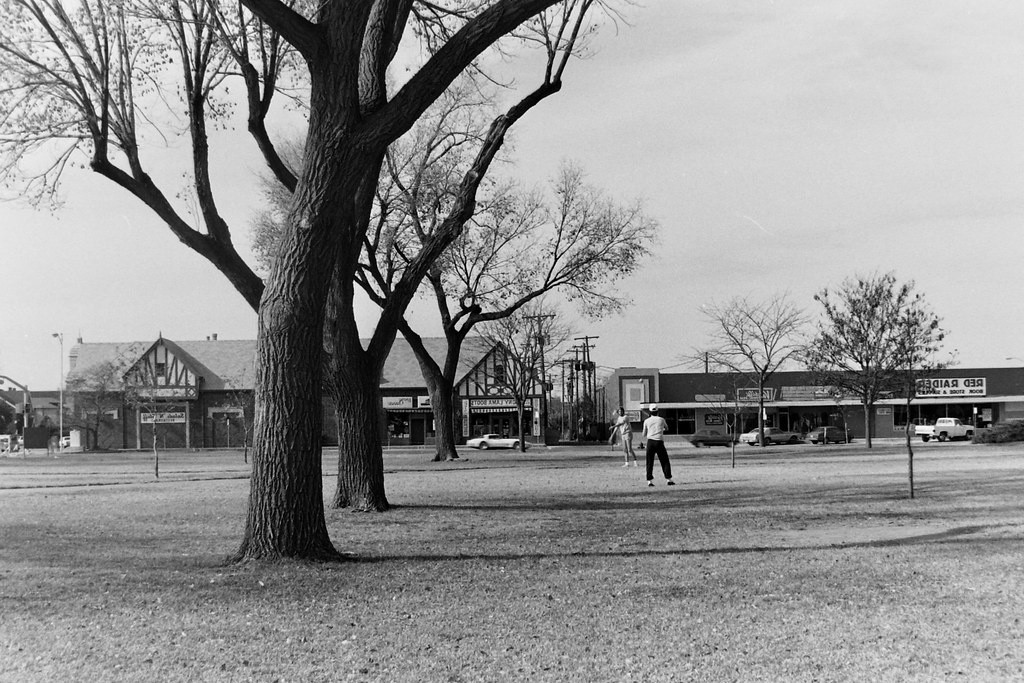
[{"left": 649, "top": 404, "right": 658, "bottom": 411}]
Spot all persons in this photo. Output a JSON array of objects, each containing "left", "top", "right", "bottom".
[
  {"left": 642, "top": 403, "right": 676, "bottom": 486},
  {"left": 609, "top": 407, "right": 638, "bottom": 467}
]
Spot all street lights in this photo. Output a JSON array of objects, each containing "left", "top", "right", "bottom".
[
  {"left": 52, "top": 332, "right": 63, "bottom": 451},
  {"left": 521, "top": 314, "right": 556, "bottom": 444},
  {"left": 545, "top": 373, "right": 558, "bottom": 413},
  {"left": 555, "top": 335, "right": 600, "bottom": 442}
]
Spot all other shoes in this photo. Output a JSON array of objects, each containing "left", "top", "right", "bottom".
[
  {"left": 667, "top": 482, "right": 675, "bottom": 485},
  {"left": 648, "top": 483, "right": 655, "bottom": 486},
  {"left": 633, "top": 461, "right": 637, "bottom": 467},
  {"left": 622, "top": 462, "right": 629, "bottom": 468}
]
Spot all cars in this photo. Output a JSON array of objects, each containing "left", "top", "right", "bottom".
[
  {"left": 59, "top": 437, "right": 70, "bottom": 448},
  {"left": 805, "top": 426, "right": 854, "bottom": 444},
  {"left": 466, "top": 434, "right": 529, "bottom": 450},
  {"left": 688, "top": 429, "right": 739, "bottom": 448},
  {"left": 740, "top": 427, "right": 802, "bottom": 446}
]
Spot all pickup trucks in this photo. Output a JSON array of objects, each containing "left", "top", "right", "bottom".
[{"left": 914, "top": 418, "right": 974, "bottom": 442}]
